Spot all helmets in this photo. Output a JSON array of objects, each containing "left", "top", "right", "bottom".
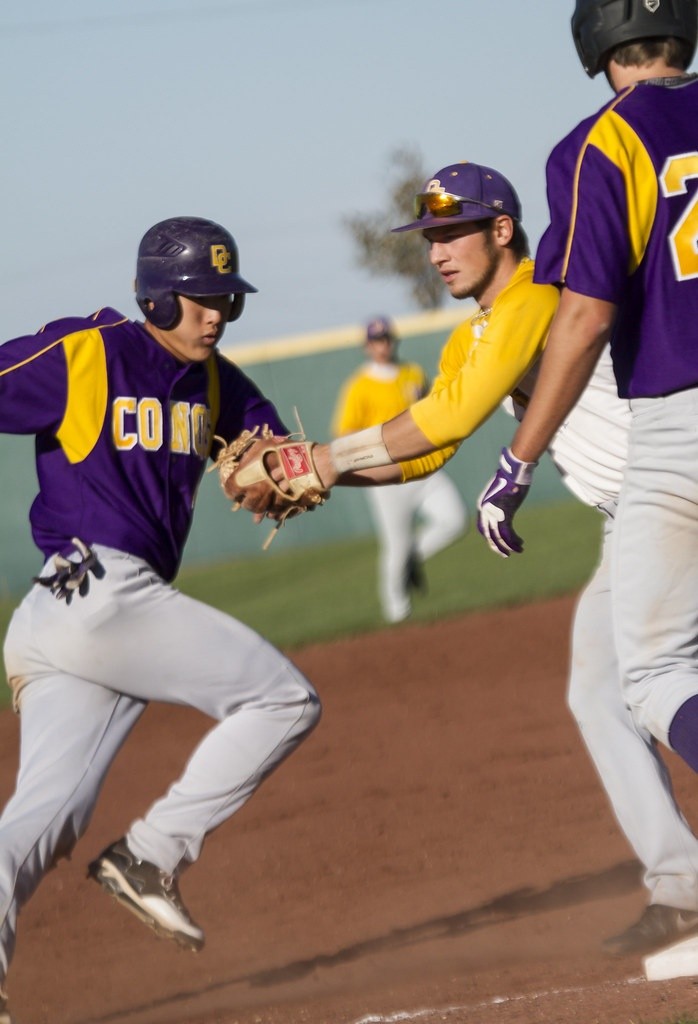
[
  {"left": 135, "top": 216, "right": 259, "bottom": 329},
  {"left": 389, "top": 163, "right": 522, "bottom": 235},
  {"left": 572, "top": 0, "right": 698, "bottom": 78}
]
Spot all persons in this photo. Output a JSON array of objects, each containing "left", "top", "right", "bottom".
[
  {"left": 0, "top": 217, "right": 335, "bottom": 1023},
  {"left": 471, "top": 1, "right": 698, "bottom": 979},
  {"left": 223, "top": 163, "right": 631, "bottom": 528},
  {"left": 335, "top": 312, "right": 471, "bottom": 631}
]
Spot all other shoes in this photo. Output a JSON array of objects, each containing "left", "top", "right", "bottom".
[{"left": 406, "top": 547, "right": 428, "bottom": 594}]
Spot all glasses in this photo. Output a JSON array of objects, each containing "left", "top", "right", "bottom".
[{"left": 412, "top": 190, "right": 518, "bottom": 221}]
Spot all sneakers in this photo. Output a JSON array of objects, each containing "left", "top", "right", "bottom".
[
  {"left": 84, "top": 835, "right": 206, "bottom": 954},
  {"left": 604, "top": 896, "right": 697, "bottom": 963}
]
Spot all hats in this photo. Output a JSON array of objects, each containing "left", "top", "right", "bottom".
[{"left": 366, "top": 319, "right": 391, "bottom": 341}]
